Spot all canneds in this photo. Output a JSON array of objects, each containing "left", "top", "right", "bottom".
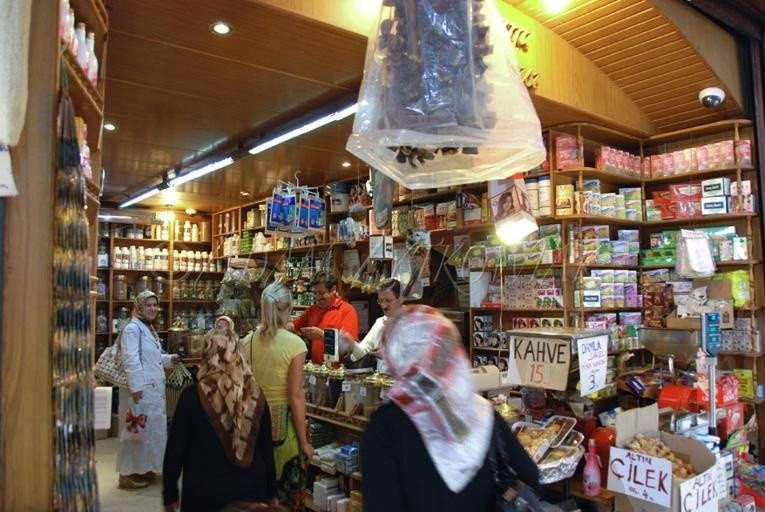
[
  {"left": 156, "top": 277, "right": 166, "bottom": 298},
  {"left": 139, "top": 275, "right": 153, "bottom": 298},
  {"left": 153, "top": 307, "right": 166, "bottom": 331},
  {"left": 117, "top": 307, "right": 128, "bottom": 331},
  {"left": 282, "top": 256, "right": 322, "bottom": 305},
  {"left": 112, "top": 275, "right": 128, "bottom": 302}
]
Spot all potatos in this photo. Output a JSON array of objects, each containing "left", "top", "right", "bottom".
[{"left": 626, "top": 431, "right": 697, "bottom": 478}]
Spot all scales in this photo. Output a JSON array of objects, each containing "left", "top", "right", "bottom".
[{"left": 673, "top": 311, "right": 721, "bottom": 453}]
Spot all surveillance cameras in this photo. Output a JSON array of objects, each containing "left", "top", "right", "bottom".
[{"left": 698, "top": 87, "right": 725, "bottom": 108}]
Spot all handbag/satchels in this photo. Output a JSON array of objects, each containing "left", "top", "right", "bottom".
[{"left": 96, "top": 346, "right": 130, "bottom": 388}]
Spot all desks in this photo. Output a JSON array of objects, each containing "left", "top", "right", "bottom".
[{"left": 554, "top": 479, "right": 613, "bottom": 512}]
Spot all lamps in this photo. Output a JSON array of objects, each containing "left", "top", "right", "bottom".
[{"left": 487, "top": 173, "right": 538, "bottom": 247}]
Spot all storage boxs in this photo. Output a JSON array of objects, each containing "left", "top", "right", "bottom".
[
  {"left": 719, "top": 451, "right": 735, "bottom": 507},
  {"left": 607, "top": 403, "right": 719, "bottom": 512}
]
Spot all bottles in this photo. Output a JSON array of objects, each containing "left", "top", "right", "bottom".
[
  {"left": 113, "top": 246, "right": 223, "bottom": 273},
  {"left": 582, "top": 438, "right": 603, "bottom": 496},
  {"left": 223, "top": 234, "right": 239, "bottom": 258},
  {"left": 161, "top": 220, "right": 200, "bottom": 241}
]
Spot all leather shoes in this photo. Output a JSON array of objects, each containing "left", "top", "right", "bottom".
[{"left": 119, "top": 475, "right": 148, "bottom": 488}]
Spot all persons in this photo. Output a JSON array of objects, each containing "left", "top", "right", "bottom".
[
  {"left": 471, "top": 316, "right": 507, "bottom": 372},
  {"left": 286, "top": 270, "right": 359, "bottom": 367},
  {"left": 359, "top": 303, "right": 542, "bottom": 510},
  {"left": 237, "top": 283, "right": 315, "bottom": 512},
  {"left": 114, "top": 290, "right": 180, "bottom": 490},
  {"left": 340, "top": 278, "right": 406, "bottom": 376},
  {"left": 160, "top": 326, "right": 287, "bottom": 511},
  {"left": 494, "top": 192, "right": 515, "bottom": 221}
]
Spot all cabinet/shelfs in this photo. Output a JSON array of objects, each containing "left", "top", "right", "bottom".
[
  {"left": 95, "top": 212, "right": 226, "bottom": 362},
  {"left": 212, "top": 175, "right": 372, "bottom": 309},
  {"left": 644, "top": 119, "right": 765, "bottom": 406},
  {"left": 0, "top": 0, "right": 108, "bottom": 512},
  {"left": 369, "top": 122, "right": 643, "bottom": 366}
]
